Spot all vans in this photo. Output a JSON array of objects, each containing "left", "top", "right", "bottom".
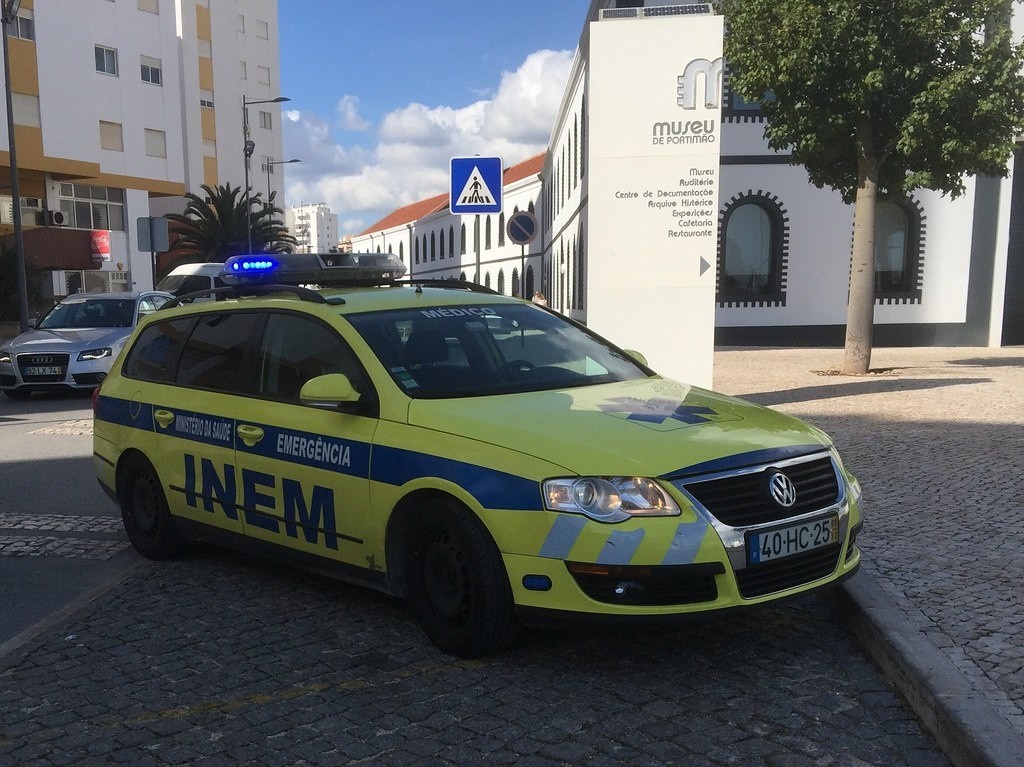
[{"left": 152, "top": 262, "right": 234, "bottom": 305}]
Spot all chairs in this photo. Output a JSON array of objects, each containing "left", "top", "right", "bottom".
[
  {"left": 395, "top": 327, "right": 494, "bottom": 399},
  {"left": 73, "top": 302, "right": 105, "bottom": 327}
]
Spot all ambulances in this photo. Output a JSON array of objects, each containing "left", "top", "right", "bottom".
[{"left": 90, "top": 254, "right": 870, "bottom": 658}]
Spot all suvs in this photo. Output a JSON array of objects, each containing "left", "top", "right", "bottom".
[{"left": 0, "top": 290, "right": 187, "bottom": 401}]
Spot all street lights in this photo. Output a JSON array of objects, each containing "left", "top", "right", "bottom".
[
  {"left": 267, "top": 159, "right": 303, "bottom": 254},
  {"left": 242, "top": 95, "right": 292, "bottom": 257},
  {"left": 1, "top": 5, "right": 29, "bottom": 335}
]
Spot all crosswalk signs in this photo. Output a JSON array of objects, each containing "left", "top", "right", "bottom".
[{"left": 449, "top": 155, "right": 503, "bottom": 214}]
